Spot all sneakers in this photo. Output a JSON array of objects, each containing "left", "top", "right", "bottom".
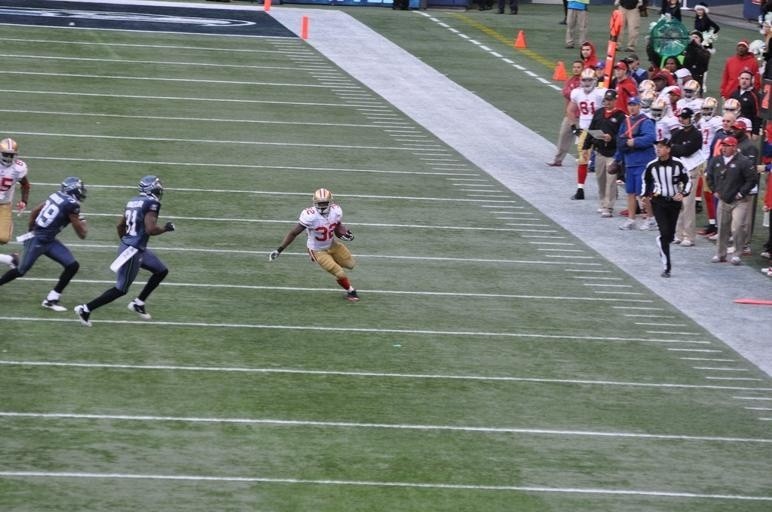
[
  {"left": 545, "top": 160, "right": 562, "bottom": 167},
  {"left": 7, "top": 253, "right": 22, "bottom": 270},
  {"left": 596, "top": 207, "right": 603, "bottom": 213},
  {"left": 74, "top": 303, "right": 93, "bottom": 327},
  {"left": 127, "top": 299, "right": 152, "bottom": 319},
  {"left": 672, "top": 202, "right": 772, "bottom": 278},
  {"left": 40, "top": 297, "right": 68, "bottom": 312},
  {"left": 617, "top": 209, "right": 659, "bottom": 233},
  {"left": 601, "top": 210, "right": 613, "bottom": 217}
]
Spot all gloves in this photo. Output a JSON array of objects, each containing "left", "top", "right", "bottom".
[
  {"left": 344, "top": 290, "right": 360, "bottom": 302},
  {"left": 15, "top": 199, "right": 27, "bottom": 217},
  {"left": 341, "top": 229, "right": 355, "bottom": 242},
  {"left": 268, "top": 249, "right": 281, "bottom": 262},
  {"left": 607, "top": 160, "right": 620, "bottom": 175},
  {"left": 571, "top": 124, "right": 583, "bottom": 137},
  {"left": 164, "top": 221, "right": 175, "bottom": 233}
]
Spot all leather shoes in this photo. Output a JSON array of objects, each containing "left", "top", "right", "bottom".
[{"left": 570, "top": 188, "right": 585, "bottom": 200}]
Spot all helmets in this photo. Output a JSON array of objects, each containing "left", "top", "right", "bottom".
[
  {"left": 579, "top": 68, "right": 597, "bottom": 91},
  {"left": 748, "top": 38, "right": 766, "bottom": 56},
  {"left": 639, "top": 78, "right": 754, "bottom": 146},
  {"left": 0, "top": 137, "right": 19, "bottom": 166},
  {"left": 138, "top": 176, "right": 165, "bottom": 204},
  {"left": 313, "top": 188, "right": 334, "bottom": 216},
  {"left": 62, "top": 176, "right": 88, "bottom": 204}
]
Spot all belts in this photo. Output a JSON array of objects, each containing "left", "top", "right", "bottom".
[{"left": 656, "top": 196, "right": 674, "bottom": 202}]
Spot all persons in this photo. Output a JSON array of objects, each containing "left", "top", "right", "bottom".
[
  {"left": 547, "top": 0, "right": 771, "bottom": 279},
  {"left": 465, "top": 0, "right": 526, "bottom": 15},
  {"left": 74, "top": 174, "right": 174, "bottom": 325},
  {"left": 1, "top": 176, "right": 87, "bottom": 311},
  {"left": 392, "top": 1, "right": 409, "bottom": 11},
  {"left": 1, "top": 252, "right": 20, "bottom": 272},
  {"left": 1, "top": 138, "right": 32, "bottom": 246},
  {"left": 269, "top": 188, "right": 361, "bottom": 303}
]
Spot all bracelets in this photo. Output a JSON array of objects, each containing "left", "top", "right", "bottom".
[{"left": 277, "top": 246, "right": 284, "bottom": 253}]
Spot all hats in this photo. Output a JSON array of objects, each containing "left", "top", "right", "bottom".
[
  {"left": 594, "top": 54, "right": 668, "bottom": 81},
  {"left": 627, "top": 96, "right": 641, "bottom": 107},
  {"left": 690, "top": 31, "right": 704, "bottom": 43},
  {"left": 605, "top": 90, "right": 618, "bottom": 101},
  {"left": 694, "top": 2, "right": 709, "bottom": 15},
  {"left": 736, "top": 39, "right": 751, "bottom": 49}
]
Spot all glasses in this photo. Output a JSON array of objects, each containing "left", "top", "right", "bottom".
[
  {"left": 1, "top": 152, "right": 17, "bottom": 160},
  {"left": 740, "top": 77, "right": 750, "bottom": 82},
  {"left": 581, "top": 78, "right": 594, "bottom": 83}
]
